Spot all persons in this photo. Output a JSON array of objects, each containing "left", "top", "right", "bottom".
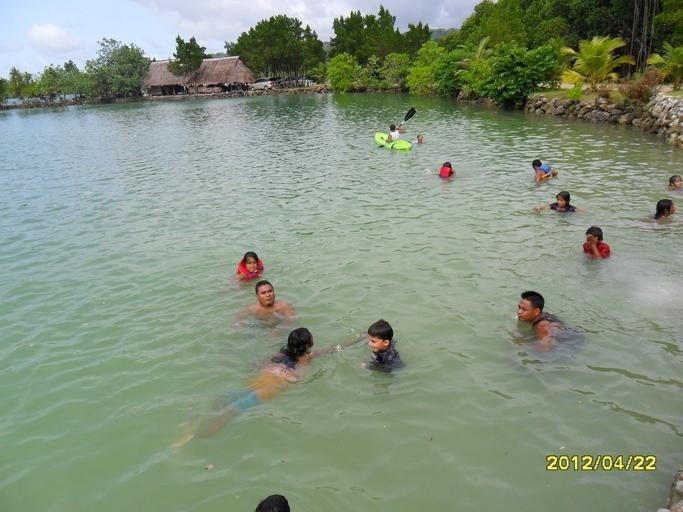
[
  {"left": 582, "top": 226, "right": 610, "bottom": 261},
  {"left": 235, "top": 280, "right": 297, "bottom": 323},
  {"left": 653, "top": 199, "right": 675, "bottom": 219},
  {"left": 386, "top": 124, "right": 400, "bottom": 143},
  {"left": 517, "top": 291, "right": 585, "bottom": 353},
  {"left": 440, "top": 162, "right": 454, "bottom": 178},
  {"left": 412, "top": 135, "right": 426, "bottom": 144},
  {"left": 363, "top": 320, "right": 405, "bottom": 372},
  {"left": 669, "top": 175, "right": 681, "bottom": 187},
  {"left": 193, "top": 328, "right": 360, "bottom": 438},
  {"left": 532, "top": 191, "right": 580, "bottom": 213},
  {"left": 255, "top": 494, "right": 290, "bottom": 512},
  {"left": 235, "top": 251, "right": 264, "bottom": 281},
  {"left": 532, "top": 159, "right": 557, "bottom": 182}
]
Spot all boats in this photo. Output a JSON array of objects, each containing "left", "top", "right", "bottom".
[{"left": 373, "top": 132, "right": 412, "bottom": 152}]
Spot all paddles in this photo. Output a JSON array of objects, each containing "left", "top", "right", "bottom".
[{"left": 399, "top": 108, "right": 416, "bottom": 127}]
